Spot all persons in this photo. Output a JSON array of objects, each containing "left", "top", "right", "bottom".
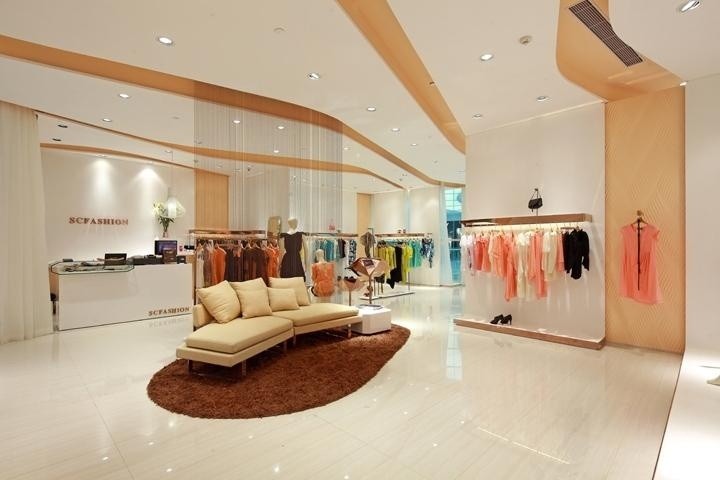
[
  {"left": 309, "top": 249, "right": 335, "bottom": 298},
  {"left": 276, "top": 217, "right": 309, "bottom": 284}
]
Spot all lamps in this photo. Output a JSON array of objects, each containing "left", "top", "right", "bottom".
[{"left": 158, "top": 148, "right": 186, "bottom": 219}]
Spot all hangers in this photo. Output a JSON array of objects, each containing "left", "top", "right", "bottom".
[
  {"left": 631, "top": 210, "right": 648, "bottom": 230},
  {"left": 461, "top": 222, "right": 583, "bottom": 237},
  {"left": 307, "top": 235, "right": 359, "bottom": 241},
  {"left": 196, "top": 235, "right": 276, "bottom": 252},
  {"left": 377, "top": 236, "right": 431, "bottom": 242}
]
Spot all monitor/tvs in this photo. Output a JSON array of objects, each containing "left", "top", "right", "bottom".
[
  {"left": 105, "top": 253, "right": 127, "bottom": 262},
  {"left": 154, "top": 240, "right": 178, "bottom": 255}
]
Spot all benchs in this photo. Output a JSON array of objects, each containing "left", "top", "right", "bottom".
[{"left": 174, "top": 276, "right": 364, "bottom": 379}]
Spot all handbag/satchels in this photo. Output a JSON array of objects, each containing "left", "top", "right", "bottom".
[{"left": 528, "top": 188, "right": 543, "bottom": 213}]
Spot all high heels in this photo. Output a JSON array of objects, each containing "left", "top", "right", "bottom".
[{"left": 489, "top": 313, "right": 512, "bottom": 326}]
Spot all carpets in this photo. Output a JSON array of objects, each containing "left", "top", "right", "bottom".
[{"left": 146, "top": 323, "right": 411, "bottom": 420}]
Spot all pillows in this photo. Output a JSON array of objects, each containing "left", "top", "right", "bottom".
[
  {"left": 266, "top": 287, "right": 301, "bottom": 312},
  {"left": 267, "top": 276, "right": 311, "bottom": 306},
  {"left": 230, "top": 278, "right": 268, "bottom": 291},
  {"left": 236, "top": 287, "right": 273, "bottom": 319},
  {"left": 195, "top": 279, "right": 241, "bottom": 324}
]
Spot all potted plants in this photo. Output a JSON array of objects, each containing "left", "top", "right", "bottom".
[{"left": 153, "top": 200, "right": 183, "bottom": 238}]
